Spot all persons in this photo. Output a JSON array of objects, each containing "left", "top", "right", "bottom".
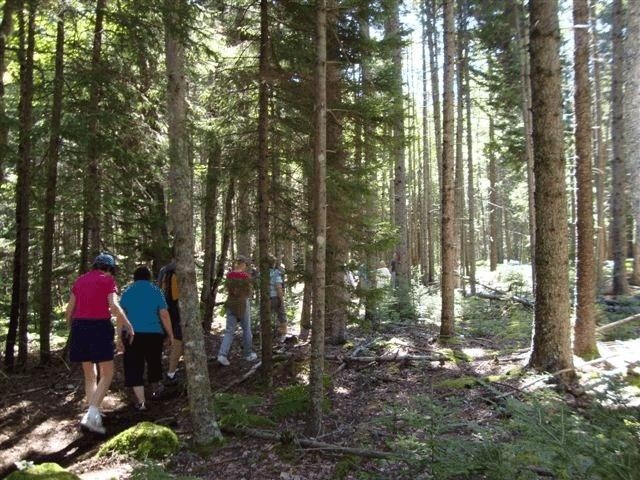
[
  {"left": 157, "top": 244, "right": 186, "bottom": 387},
  {"left": 260, "top": 252, "right": 288, "bottom": 350},
  {"left": 391, "top": 250, "right": 399, "bottom": 292},
  {"left": 115, "top": 266, "right": 176, "bottom": 412},
  {"left": 65, "top": 252, "right": 135, "bottom": 437},
  {"left": 218, "top": 253, "right": 255, "bottom": 368},
  {"left": 376, "top": 260, "right": 391, "bottom": 278}
]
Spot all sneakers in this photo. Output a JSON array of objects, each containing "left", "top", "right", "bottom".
[
  {"left": 137, "top": 402, "right": 150, "bottom": 410},
  {"left": 217, "top": 356, "right": 230, "bottom": 366},
  {"left": 152, "top": 389, "right": 166, "bottom": 398},
  {"left": 80, "top": 412, "right": 106, "bottom": 433},
  {"left": 246, "top": 353, "right": 257, "bottom": 361},
  {"left": 282, "top": 334, "right": 290, "bottom": 344},
  {"left": 164, "top": 377, "right": 176, "bottom": 384}
]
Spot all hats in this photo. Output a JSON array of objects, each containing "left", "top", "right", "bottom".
[{"left": 95, "top": 255, "right": 115, "bottom": 265}]
